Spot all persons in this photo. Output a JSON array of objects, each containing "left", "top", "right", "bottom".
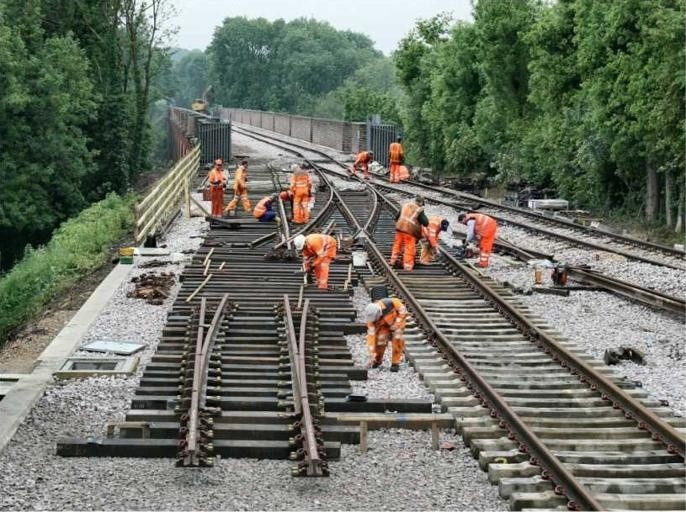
[
  {"left": 458, "top": 213, "right": 497, "bottom": 267},
  {"left": 352, "top": 151, "right": 373, "bottom": 180},
  {"left": 207, "top": 160, "right": 224, "bottom": 218},
  {"left": 290, "top": 164, "right": 313, "bottom": 224},
  {"left": 223, "top": 161, "right": 253, "bottom": 217},
  {"left": 390, "top": 197, "right": 428, "bottom": 271},
  {"left": 364, "top": 298, "right": 407, "bottom": 372},
  {"left": 253, "top": 193, "right": 278, "bottom": 222},
  {"left": 294, "top": 234, "right": 336, "bottom": 289},
  {"left": 280, "top": 190, "right": 293, "bottom": 222},
  {"left": 418, "top": 216, "right": 449, "bottom": 266},
  {"left": 400, "top": 166, "right": 410, "bottom": 181},
  {"left": 387, "top": 136, "right": 404, "bottom": 183}
]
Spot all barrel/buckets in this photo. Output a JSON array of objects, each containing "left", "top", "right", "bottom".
[{"left": 352, "top": 251, "right": 368, "bottom": 267}]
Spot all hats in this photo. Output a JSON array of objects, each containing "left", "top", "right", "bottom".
[
  {"left": 215, "top": 159, "right": 223, "bottom": 165},
  {"left": 442, "top": 221, "right": 449, "bottom": 231},
  {"left": 416, "top": 198, "right": 425, "bottom": 206}
]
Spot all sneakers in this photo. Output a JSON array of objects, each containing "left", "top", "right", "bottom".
[{"left": 390, "top": 364, "right": 400, "bottom": 372}]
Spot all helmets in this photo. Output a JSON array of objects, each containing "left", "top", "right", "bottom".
[
  {"left": 365, "top": 303, "right": 378, "bottom": 322},
  {"left": 280, "top": 192, "right": 287, "bottom": 200},
  {"left": 294, "top": 235, "right": 305, "bottom": 251}
]
